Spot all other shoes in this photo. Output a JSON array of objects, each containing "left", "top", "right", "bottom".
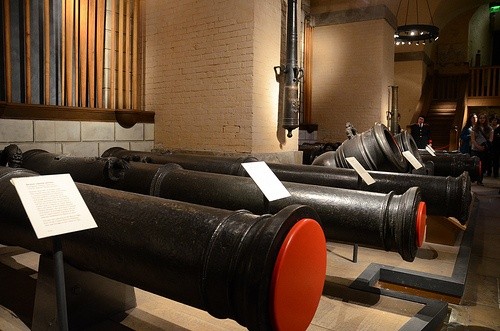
[
  {"left": 477, "top": 180, "right": 485, "bottom": 186},
  {"left": 469, "top": 179, "right": 475, "bottom": 184}
]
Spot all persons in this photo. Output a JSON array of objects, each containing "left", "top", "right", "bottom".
[
  {"left": 460, "top": 113, "right": 478, "bottom": 154},
  {"left": 411, "top": 114, "right": 433, "bottom": 149},
  {"left": 490, "top": 115, "right": 500, "bottom": 177},
  {"left": 397, "top": 114, "right": 401, "bottom": 135},
  {"left": 471, "top": 112, "right": 494, "bottom": 178}
]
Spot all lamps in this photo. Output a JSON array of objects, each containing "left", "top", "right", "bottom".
[{"left": 393, "top": 0, "right": 440, "bottom": 46}]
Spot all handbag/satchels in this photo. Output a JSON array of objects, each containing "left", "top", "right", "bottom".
[{"left": 480, "top": 140, "right": 492, "bottom": 152}]
